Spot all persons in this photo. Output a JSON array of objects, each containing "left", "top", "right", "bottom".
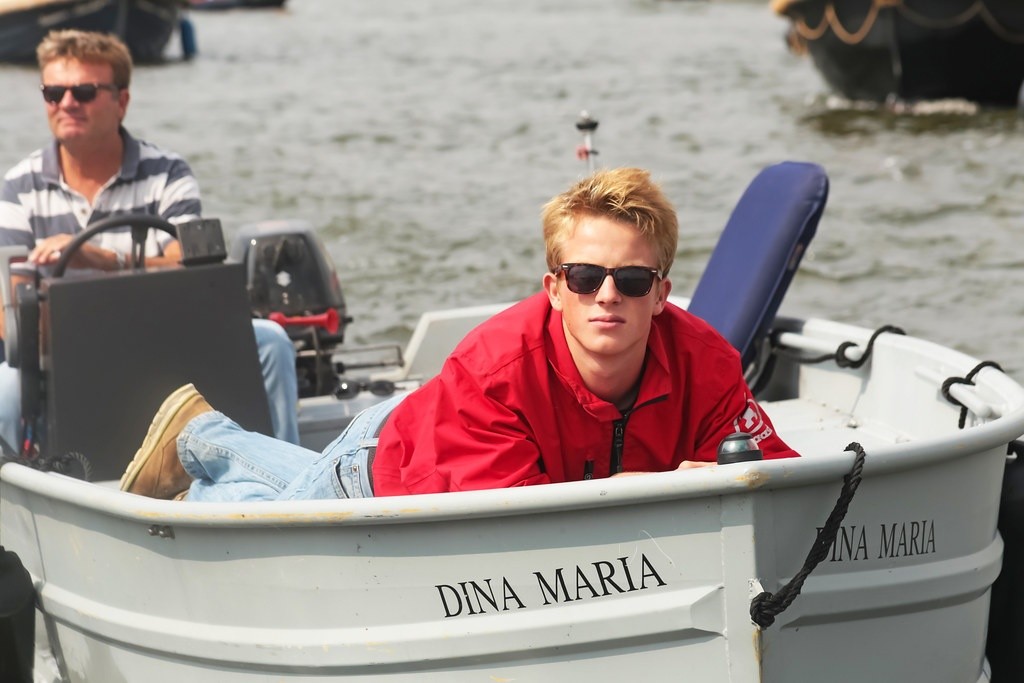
[
  {"left": 0, "top": 27, "right": 298, "bottom": 463},
  {"left": 116, "top": 167, "right": 804, "bottom": 505}
]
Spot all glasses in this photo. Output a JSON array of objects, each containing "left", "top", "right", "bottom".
[
  {"left": 334, "top": 380, "right": 407, "bottom": 400},
  {"left": 553, "top": 262, "right": 663, "bottom": 297},
  {"left": 42, "top": 83, "right": 119, "bottom": 103}
]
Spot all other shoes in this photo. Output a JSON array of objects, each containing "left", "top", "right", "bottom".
[{"left": 118, "top": 382, "right": 216, "bottom": 501}]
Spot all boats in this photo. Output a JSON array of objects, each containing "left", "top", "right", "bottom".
[{"left": 0, "top": 112, "right": 1023, "bottom": 681}]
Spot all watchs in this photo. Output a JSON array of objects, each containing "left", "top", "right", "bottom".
[{"left": 114, "top": 248, "right": 128, "bottom": 271}]
